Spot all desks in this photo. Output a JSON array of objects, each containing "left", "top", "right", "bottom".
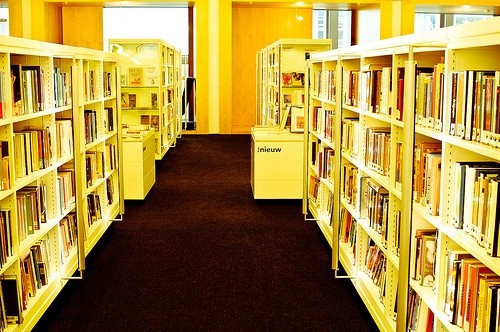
[
  {"left": 122, "top": 128, "right": 156, "bottom": 200},
  {"left": 250, "top": 127, "right": 304, "bottom": 199}
]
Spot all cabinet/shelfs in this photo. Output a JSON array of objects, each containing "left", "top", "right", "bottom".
[
  {"left": 0, "top": 36, "right": 124, "bottom": 332},
  {"left": 108, "top": 39, "right": 182, "bottom": 160},
  {"left": 259, "top": 38, "right": 332, "bottom": 130},
  {"left": 303, "top": 16, "right": 500, "bottom": 332}
]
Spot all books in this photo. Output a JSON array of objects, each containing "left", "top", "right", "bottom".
[
  {"left": 415, "top": 62, "right": 444, "bottom": 133},
  {"left": 313, "top": 67, "right": 404, "bottom": 121},
  {"left": 85, "top": 70, "right": 102, "bottom": 227},
  {"left": 452, "top": 161, "right": 500, "bottom": 257},
  {"left": 0, "top": 186, "right": 48, "bottom": 332},
  {"left": 54, "top": 66, "right": 78, "bottom": 259},
  {"left": 340, "top": 118, "right": 403, "bottom": 320},
  {"left": 449, "top": 70, "right": 500, "bottom": 149},
  {"left": 121, "top": 65, "right": 159, "bottom": 86},
  {"left": 290, "top": 106, "right": 305, "bottom": 133},
  {"left": 282, "top": 72, "right": 305, "bottom": 87},
  {"left": 413, "top": 141, "right": 442, "bottom": 216},
  {"left": 412, "top": 227, "right": 439, "bottom": 287},
  {"left": 0, "top": 64, "right": 52, "bottom": 191},
  {"left": 121, "top": 92, "right": 158, "bottom": 109},
  {"left": 444, "top": 250, "right": 500, "bottom": 332},
  {"left": 122, "top": 114, "right": 159, "bottom": 137},
  {"left": 407, "top": 290, "right": 435, "bottom": 332},
  {"left": 103, "top": 71, "right": 117, "bottom": 205},
  {"left": 308, "top": 106, "right": 334, "bottom": 229}
]
